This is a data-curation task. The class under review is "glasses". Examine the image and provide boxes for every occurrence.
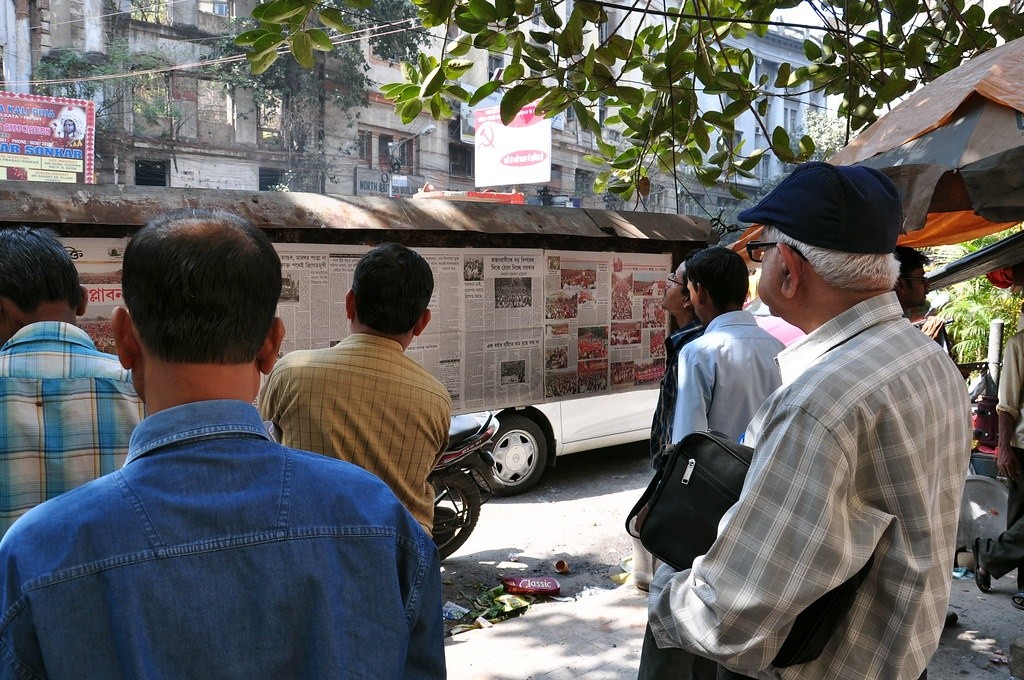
[745,240,810,265]
[899,277,928,284]
[664,272,687,289]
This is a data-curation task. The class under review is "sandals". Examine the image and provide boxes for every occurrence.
[970,536,993,594]
[1011,592,1024,611]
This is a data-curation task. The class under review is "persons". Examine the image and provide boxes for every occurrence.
[650,261,705,470]
[650,248,785,576]
[63,117,77,138]
[971,303,1024,611]
[258,244,451,542]
[892,244,929,314]
[0,226,146,547]
[0,209,449,680]
[637,162,972,680]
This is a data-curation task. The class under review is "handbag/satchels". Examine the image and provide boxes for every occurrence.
[623,429,874,669]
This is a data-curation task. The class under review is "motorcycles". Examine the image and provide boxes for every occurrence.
[969,367,1001,480]
[419,411,502,563]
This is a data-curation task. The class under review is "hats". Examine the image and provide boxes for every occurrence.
[736,164,905,254]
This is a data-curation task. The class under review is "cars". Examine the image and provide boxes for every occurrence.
[445,389,661,497]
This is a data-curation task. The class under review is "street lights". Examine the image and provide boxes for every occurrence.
[389,124,437,198]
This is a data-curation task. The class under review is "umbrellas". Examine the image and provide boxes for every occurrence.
[726,33,1024,269]
